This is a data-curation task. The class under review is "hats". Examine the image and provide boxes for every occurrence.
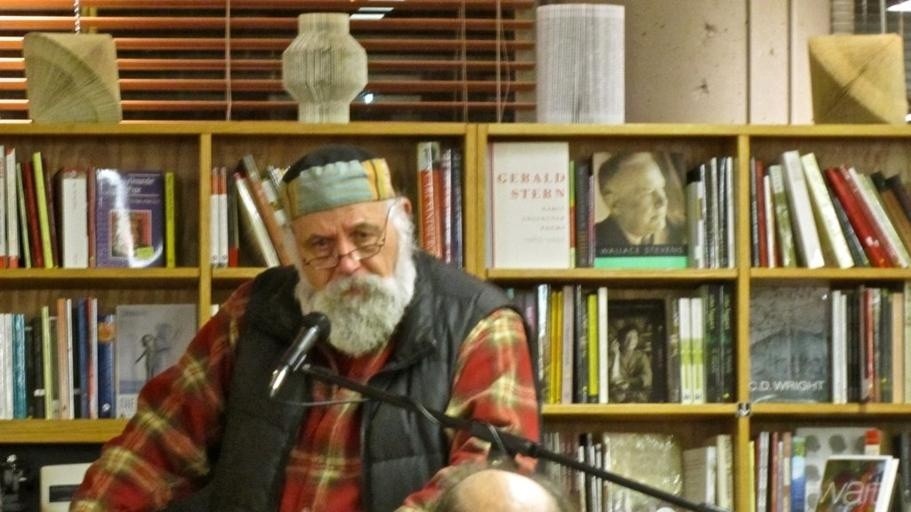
[276,145,396,223]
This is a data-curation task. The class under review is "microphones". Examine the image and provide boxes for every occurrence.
[265,308,331,399]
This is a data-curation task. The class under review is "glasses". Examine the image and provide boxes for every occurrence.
[289,202,399,272]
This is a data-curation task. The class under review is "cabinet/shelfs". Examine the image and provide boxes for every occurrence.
[1,122,910,510]
